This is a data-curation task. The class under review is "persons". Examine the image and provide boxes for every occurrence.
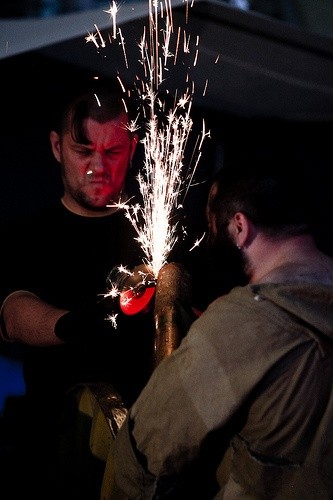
[101,143,333,500]
[0,69,202,500]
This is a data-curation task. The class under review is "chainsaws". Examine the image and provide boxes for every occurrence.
[106,264,157,316]
